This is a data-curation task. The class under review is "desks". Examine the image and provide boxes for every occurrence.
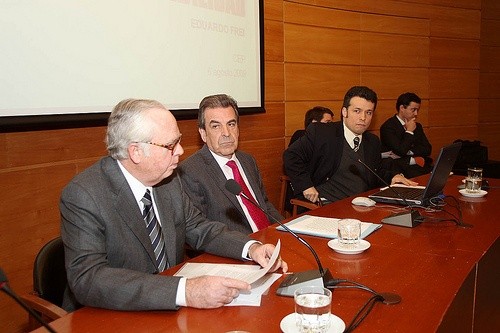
[31,173,500,333]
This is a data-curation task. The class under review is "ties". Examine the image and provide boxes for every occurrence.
[141,189,170,273]
[353,137,360,153]
[226,159,270,230]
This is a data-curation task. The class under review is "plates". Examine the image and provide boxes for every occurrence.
[327,237,371,254]
[459,189,488,197]
[280,311,346,333]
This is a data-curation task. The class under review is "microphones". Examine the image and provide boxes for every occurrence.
[224,178,335,296]
[351,154,421,228]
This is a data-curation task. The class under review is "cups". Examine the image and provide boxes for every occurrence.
[465,167,483,194]
[337,218,362,251]
[294,286,333,333]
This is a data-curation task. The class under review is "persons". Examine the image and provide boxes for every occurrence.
[58,99,288,313]
[282,85,419,216]
[305,106,334,134]
[380,92,434,177]
[179,94,284,257]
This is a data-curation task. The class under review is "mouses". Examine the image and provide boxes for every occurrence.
[351,196,377,207]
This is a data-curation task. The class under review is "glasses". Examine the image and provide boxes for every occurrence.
[132,134,182,156]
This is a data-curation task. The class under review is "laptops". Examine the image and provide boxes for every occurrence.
[368,141,463,207]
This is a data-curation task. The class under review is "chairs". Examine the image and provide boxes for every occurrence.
[279,175,320,218]
[21,237,68,330]
